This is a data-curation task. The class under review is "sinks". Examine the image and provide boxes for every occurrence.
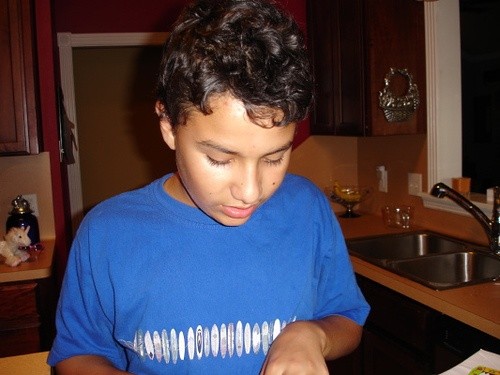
[399,251,500,283]
[345,234,472,261]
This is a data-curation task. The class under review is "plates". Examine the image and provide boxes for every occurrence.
[437,348,500,375]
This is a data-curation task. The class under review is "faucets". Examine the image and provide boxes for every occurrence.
[430,182,492,236]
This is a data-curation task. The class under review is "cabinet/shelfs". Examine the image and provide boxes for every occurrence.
[307,0,425,137]
[0,0,63,151]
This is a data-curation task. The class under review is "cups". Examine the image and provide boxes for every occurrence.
[382,206,396,232]
[396,207,413,229]
